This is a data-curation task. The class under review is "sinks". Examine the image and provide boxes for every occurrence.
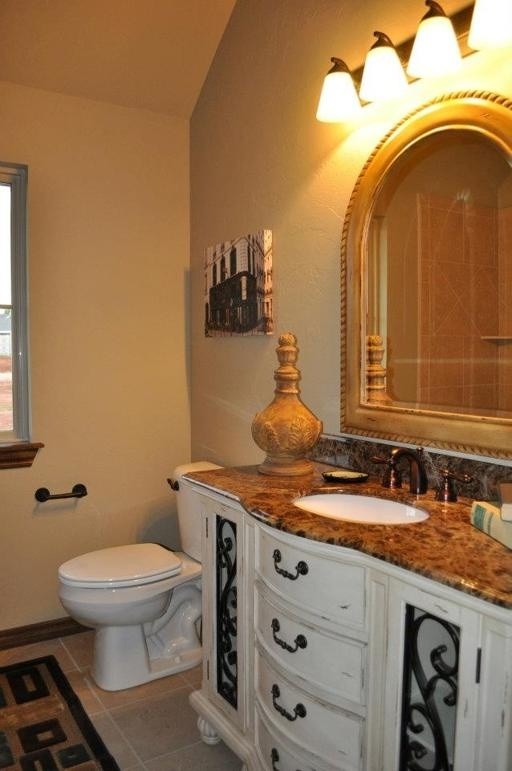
[289,488,430,524]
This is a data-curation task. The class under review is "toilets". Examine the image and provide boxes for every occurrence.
[58,462,225,693]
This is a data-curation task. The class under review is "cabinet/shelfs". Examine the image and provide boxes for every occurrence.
[177,465,512,771]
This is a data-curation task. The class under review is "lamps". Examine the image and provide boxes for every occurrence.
[314,0,511,125]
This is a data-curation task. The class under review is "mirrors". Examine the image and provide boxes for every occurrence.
[339,90,511,460]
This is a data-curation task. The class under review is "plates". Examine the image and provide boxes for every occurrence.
[320,469,370,483]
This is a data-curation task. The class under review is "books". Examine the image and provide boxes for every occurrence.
[497,482,511,522]
[469,500,512,550]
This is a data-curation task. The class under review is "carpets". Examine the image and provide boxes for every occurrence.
[1,655,124,771]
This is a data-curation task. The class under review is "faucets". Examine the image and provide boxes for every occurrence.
[387,447,428,494]
